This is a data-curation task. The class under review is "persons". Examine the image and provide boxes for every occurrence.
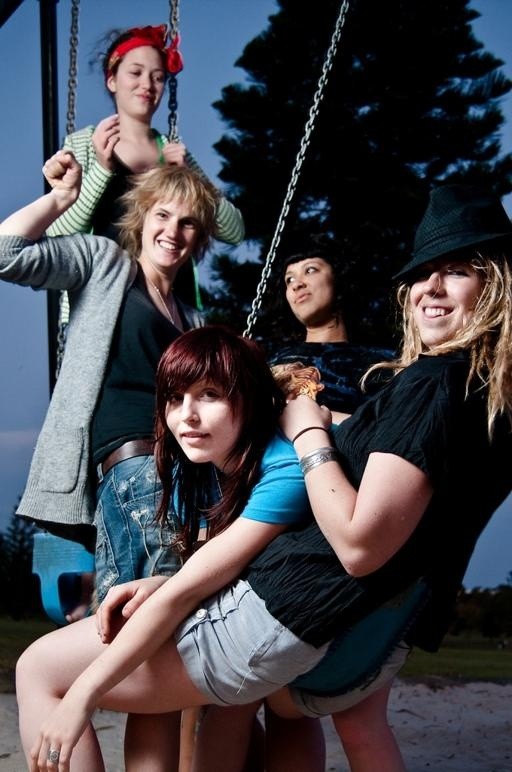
[1,147,226,771]
[200,241,404,772]
[43,25,246,343]
[31,325,413,770]
[15,170,511,772]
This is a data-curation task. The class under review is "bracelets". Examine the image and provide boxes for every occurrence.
[298,446,337,476]
[292,425,327,445]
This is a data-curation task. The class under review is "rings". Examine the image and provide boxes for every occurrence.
[46,749,59,765]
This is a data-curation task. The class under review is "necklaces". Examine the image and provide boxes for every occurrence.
[143,272,178,327]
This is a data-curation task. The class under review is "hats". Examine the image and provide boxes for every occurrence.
[390,174,511,284]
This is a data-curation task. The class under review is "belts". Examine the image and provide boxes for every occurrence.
[96,439,157,477]
